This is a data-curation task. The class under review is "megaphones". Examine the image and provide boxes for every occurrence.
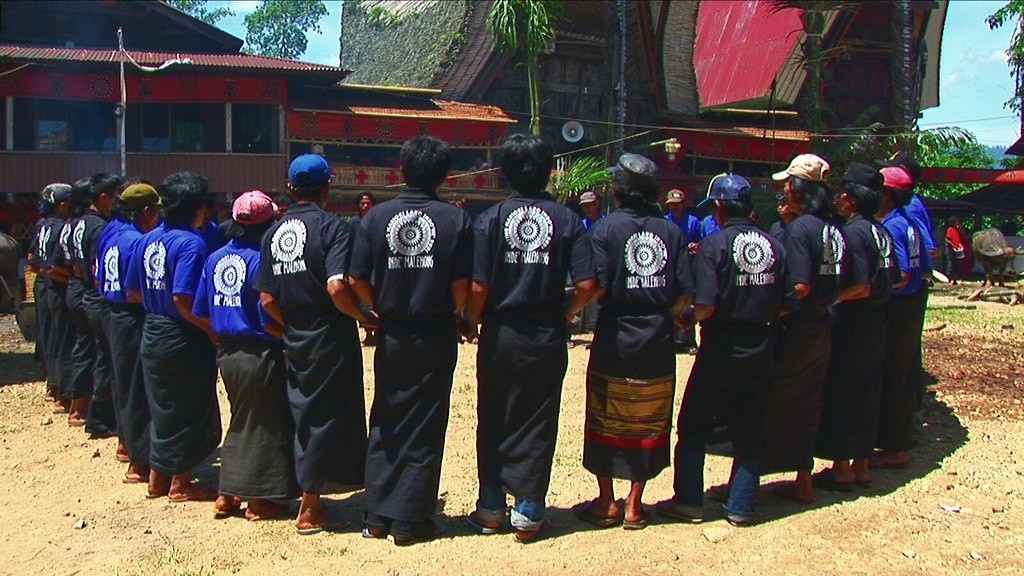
[562,120,584,143]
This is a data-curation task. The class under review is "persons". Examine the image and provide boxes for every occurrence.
[254,151,382,533]
[469,133,599,544]
[348,133,474,548]
[654,172,802,521]
[26,168,298,522]
[569,152,694,530]
[703,152,969,506]
[351,187,702,356]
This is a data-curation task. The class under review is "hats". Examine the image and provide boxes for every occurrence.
[874,151,920,180]
[665,189,685,204]
[578,191,601,205]
[878,166,913,192]
[771,153,832,187]
[120,183,163,209]
[289,153,338,188]
[696,172,752,212]
[605,153,658,193]
[832,162,884,192]
[232,190,279,225]
[38,183,72,215]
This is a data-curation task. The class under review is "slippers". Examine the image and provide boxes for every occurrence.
[45,397,909,547]
